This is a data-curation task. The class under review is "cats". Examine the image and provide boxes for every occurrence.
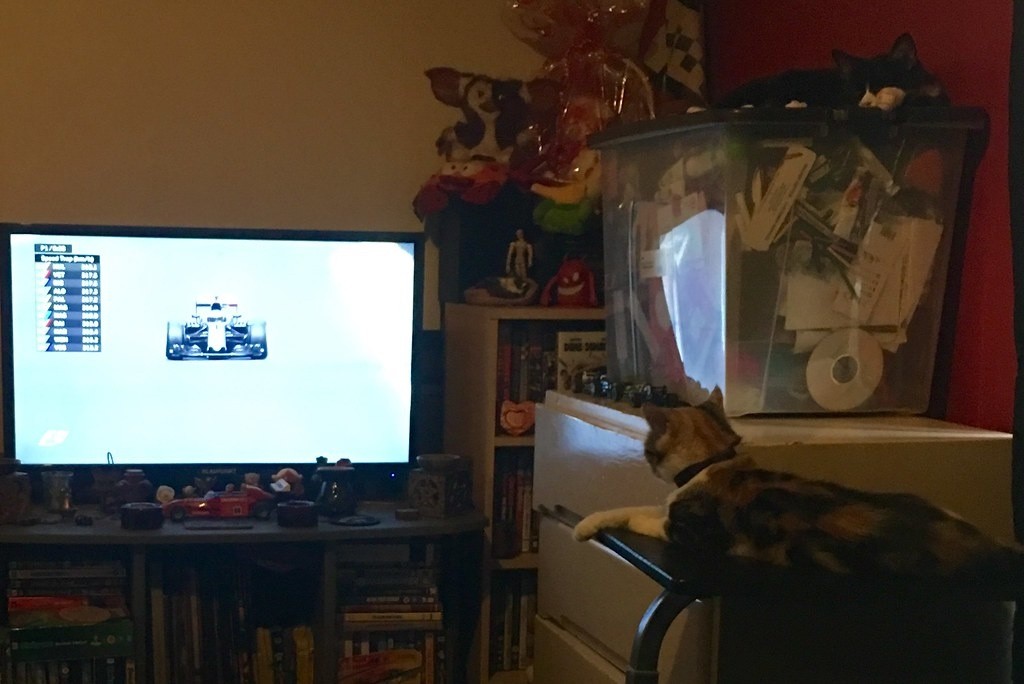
[572,386,1024,601]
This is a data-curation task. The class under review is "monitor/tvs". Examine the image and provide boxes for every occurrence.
[0,223,427,495]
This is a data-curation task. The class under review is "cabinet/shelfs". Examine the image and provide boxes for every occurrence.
[0,497,487,684]
[438,298,623,684]
[520,385,1019,683]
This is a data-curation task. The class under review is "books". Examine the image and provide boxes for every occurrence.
[0,562,442,684]
[489,320,608,671]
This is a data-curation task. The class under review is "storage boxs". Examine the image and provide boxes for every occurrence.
[586,106,990,410]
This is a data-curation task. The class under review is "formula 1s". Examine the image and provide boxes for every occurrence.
[161,483,279,520]
[165,299,269,361]
